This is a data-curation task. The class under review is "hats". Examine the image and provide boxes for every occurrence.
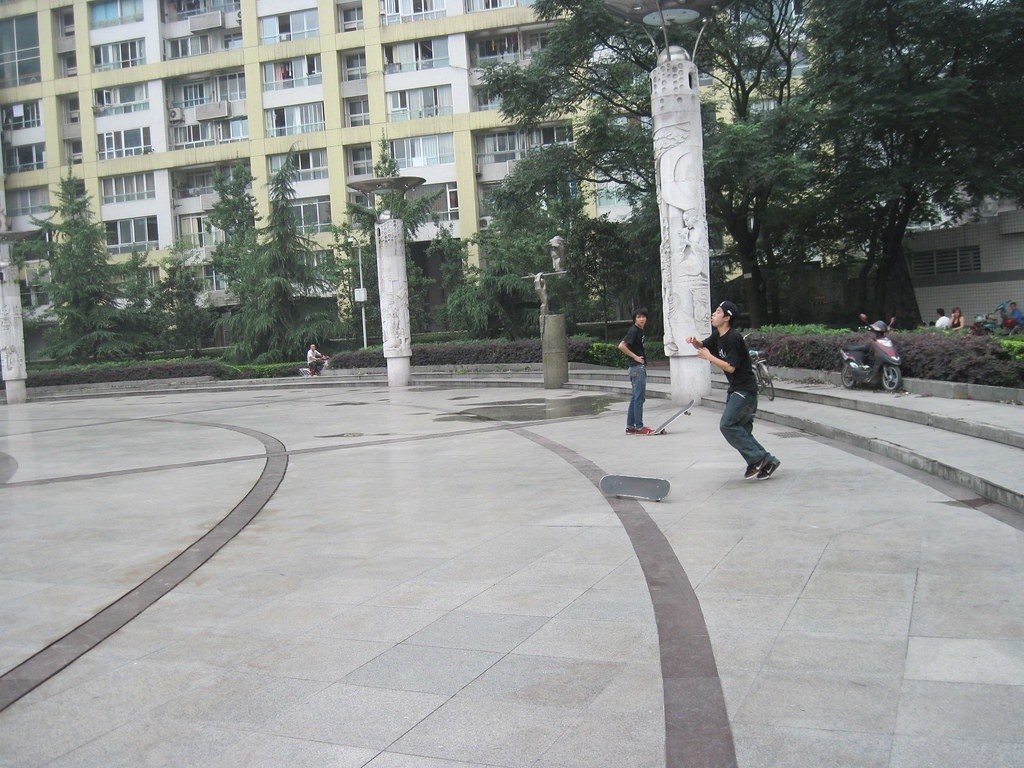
[720,301,740,318]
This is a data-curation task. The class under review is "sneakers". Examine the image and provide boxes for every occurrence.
[743,457,765,480]
[635,426,656,436]
[755,456,781,480]
[625,427,635,435]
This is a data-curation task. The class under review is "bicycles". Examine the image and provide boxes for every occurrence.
[721,334,776,401]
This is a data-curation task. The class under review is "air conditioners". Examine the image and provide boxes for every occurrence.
[168,108,185,122]
[0,271,7,283]
[479,216,492,231]
[236,10,242,21]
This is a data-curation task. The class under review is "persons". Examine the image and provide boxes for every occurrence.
[618,306,655,435]
[935,308,949,329]
[306,344,329,375]
[686,300,781,480]
[929,321,935,325]
[1010,302,1024,325]
[948,306,965,330]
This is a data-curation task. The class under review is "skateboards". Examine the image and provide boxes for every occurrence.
[654,399,695,435]
[598,475,672,502]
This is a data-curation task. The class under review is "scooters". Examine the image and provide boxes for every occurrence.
[299,354,330,377]
[970,300,1024,339]
[840,313,902,391]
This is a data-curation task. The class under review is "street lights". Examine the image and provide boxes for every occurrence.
[347,176,427,388]
[603,0,732,409]
[342,229,369,352]
